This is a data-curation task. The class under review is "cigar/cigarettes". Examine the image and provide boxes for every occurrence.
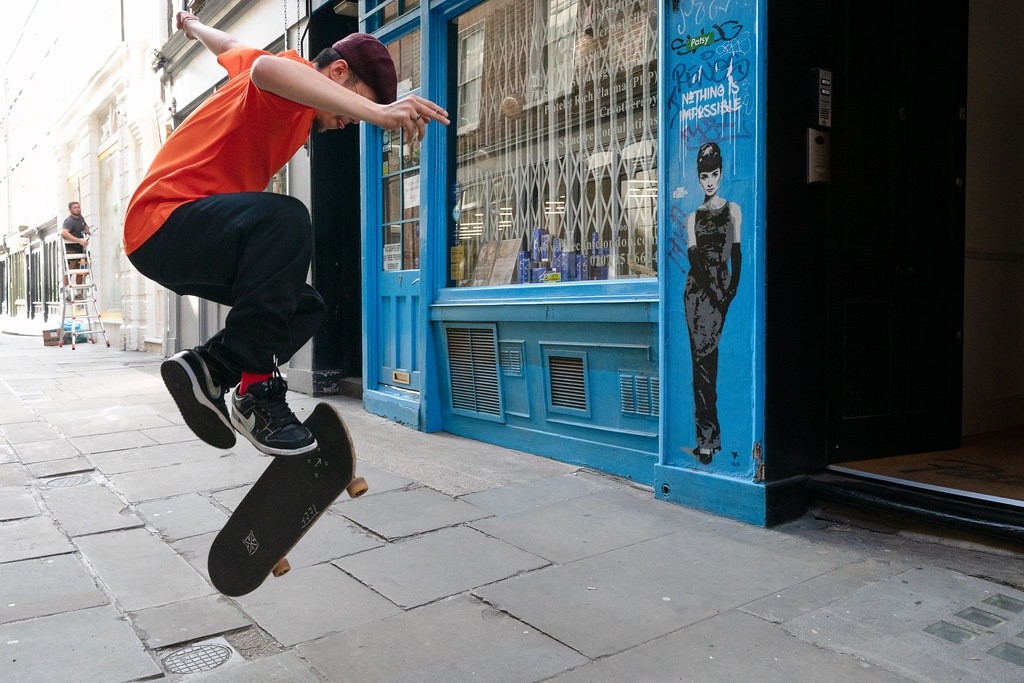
[435,110,440,114]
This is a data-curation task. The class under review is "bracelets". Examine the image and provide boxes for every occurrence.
[181,16,200,41]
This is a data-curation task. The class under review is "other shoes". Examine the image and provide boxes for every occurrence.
[692,446,712,465]
[66,295,71,301]
[74,294,86,300]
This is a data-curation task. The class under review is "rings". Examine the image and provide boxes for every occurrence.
[412,113,422,122]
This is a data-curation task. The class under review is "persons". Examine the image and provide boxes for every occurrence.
[682,143,743,474]
[61,200,92,303]
[123,11,451,458]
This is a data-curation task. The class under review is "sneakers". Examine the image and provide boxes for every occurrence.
[229,366,319,457]
[161,343,237,450]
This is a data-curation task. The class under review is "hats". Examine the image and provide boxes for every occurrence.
[332,32,397,105]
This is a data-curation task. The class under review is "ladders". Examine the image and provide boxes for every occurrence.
[58,232,110,350]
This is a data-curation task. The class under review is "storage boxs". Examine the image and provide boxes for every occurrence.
[43,329,62,346]
